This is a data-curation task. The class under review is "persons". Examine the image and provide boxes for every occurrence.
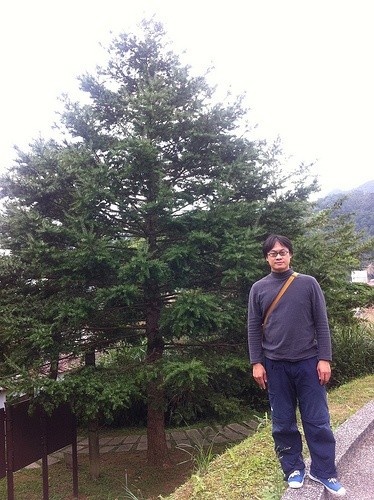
[247,235,346,497]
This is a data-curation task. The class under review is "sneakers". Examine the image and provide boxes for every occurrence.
[308,470,346,496]
[288,469,306,488]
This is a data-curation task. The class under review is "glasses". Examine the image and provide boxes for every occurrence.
[267,250,288,256]
